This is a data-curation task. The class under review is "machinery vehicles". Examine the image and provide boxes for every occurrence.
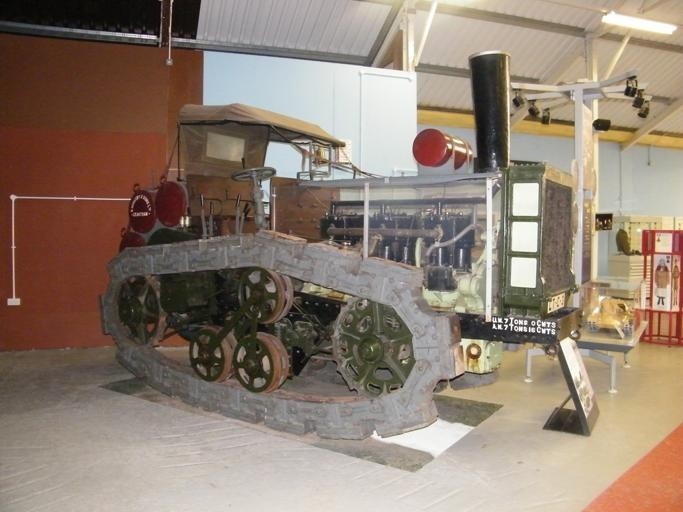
[99,50,583,446]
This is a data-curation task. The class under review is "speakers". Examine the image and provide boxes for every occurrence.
[593,119,611,131]
